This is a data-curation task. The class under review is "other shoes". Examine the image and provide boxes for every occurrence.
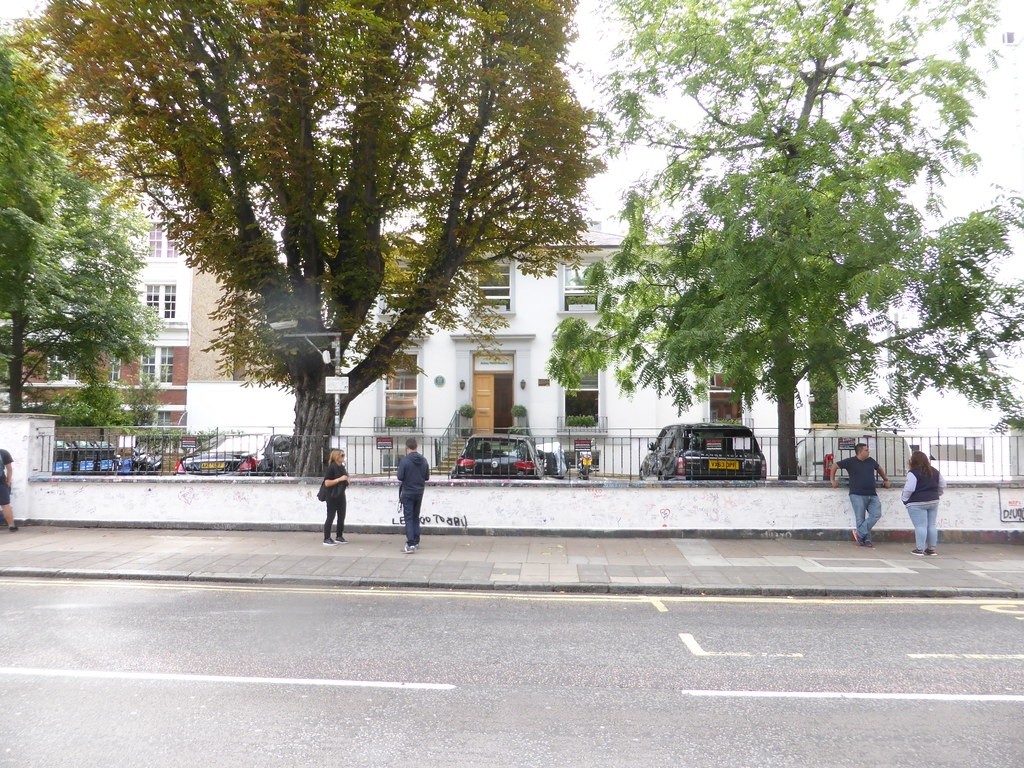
[9,525,18,532]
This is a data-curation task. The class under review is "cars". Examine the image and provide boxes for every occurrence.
[638,423,767,481]
[172,434,293,476]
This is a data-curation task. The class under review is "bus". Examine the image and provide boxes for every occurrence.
[450,434,545,480]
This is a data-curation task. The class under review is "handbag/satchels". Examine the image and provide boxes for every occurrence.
[317,481,329,501]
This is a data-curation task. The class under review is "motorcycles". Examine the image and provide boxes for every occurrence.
[132,442,163,475]
[575,438,595,480]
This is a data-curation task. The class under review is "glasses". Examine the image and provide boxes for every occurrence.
[339,454,345,457]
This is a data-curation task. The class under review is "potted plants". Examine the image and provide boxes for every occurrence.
[508,405,527,434]
[459,405,476,436]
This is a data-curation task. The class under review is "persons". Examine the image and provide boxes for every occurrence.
[397,437,430,553]
[901,451,947,556]
[830,443,890,547]
[0,449,18,532]
[323,449,350,546]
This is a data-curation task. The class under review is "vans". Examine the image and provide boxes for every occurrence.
[796,424,911,479]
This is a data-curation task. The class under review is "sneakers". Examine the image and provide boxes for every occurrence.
[924,548,937,555]
[852,529,865,546]
[911,548,924,556]
[413,544,419,552]
[400,545,415,554]
[862,537,873,547]
[323,538,338,546]
[334,536,349,544]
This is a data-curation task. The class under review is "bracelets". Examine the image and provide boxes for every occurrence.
[884,478,888,481]
[336,478,339,483]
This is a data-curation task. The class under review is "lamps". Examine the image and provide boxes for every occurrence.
[460,380,465,390]
[521,379,526,389]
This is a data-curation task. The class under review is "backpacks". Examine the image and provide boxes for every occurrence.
[0,456,4,481]
